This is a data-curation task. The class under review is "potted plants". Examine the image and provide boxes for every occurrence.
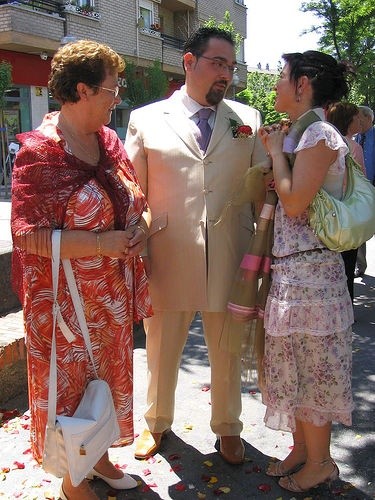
[77,4,99,18]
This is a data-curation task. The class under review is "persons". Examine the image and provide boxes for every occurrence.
[9,39,152,500]
[245,51,354,491]
[261,102,374,304]
[120,26,271,463]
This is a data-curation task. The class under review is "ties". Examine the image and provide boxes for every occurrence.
[193,109,212,156]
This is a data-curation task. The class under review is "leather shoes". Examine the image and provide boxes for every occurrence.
[134,429,163,459]
[218,435,246,464]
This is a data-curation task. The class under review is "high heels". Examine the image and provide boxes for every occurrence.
[86,467,137,489]
[278,457,340,493]
[59,483,99,500]
[266,443,307,476]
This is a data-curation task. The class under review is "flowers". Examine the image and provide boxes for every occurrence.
[230,119,253,142]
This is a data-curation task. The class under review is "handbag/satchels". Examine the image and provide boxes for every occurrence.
[305,156,375,253]
[42,379,121,488]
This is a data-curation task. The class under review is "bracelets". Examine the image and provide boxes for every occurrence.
[95,232,100,257]
[137,225,146,236]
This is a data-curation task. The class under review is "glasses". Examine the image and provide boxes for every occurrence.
[92,84,119,97]
[197,54,239,73]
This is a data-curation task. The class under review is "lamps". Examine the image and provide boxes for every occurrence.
[40,50,47,60]
